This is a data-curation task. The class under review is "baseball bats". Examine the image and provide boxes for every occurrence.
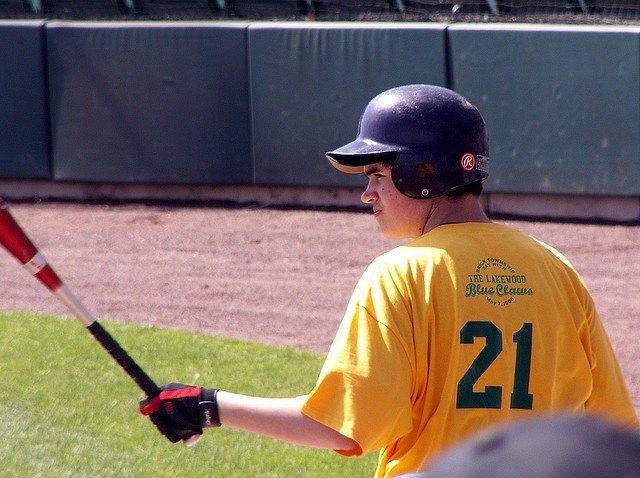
[0,195,202,448]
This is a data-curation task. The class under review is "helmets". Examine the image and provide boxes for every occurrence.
[325,83,490,198]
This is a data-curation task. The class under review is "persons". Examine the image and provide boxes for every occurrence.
[139,83,640,478]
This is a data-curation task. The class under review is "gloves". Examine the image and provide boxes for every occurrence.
[138,383,222,443]
